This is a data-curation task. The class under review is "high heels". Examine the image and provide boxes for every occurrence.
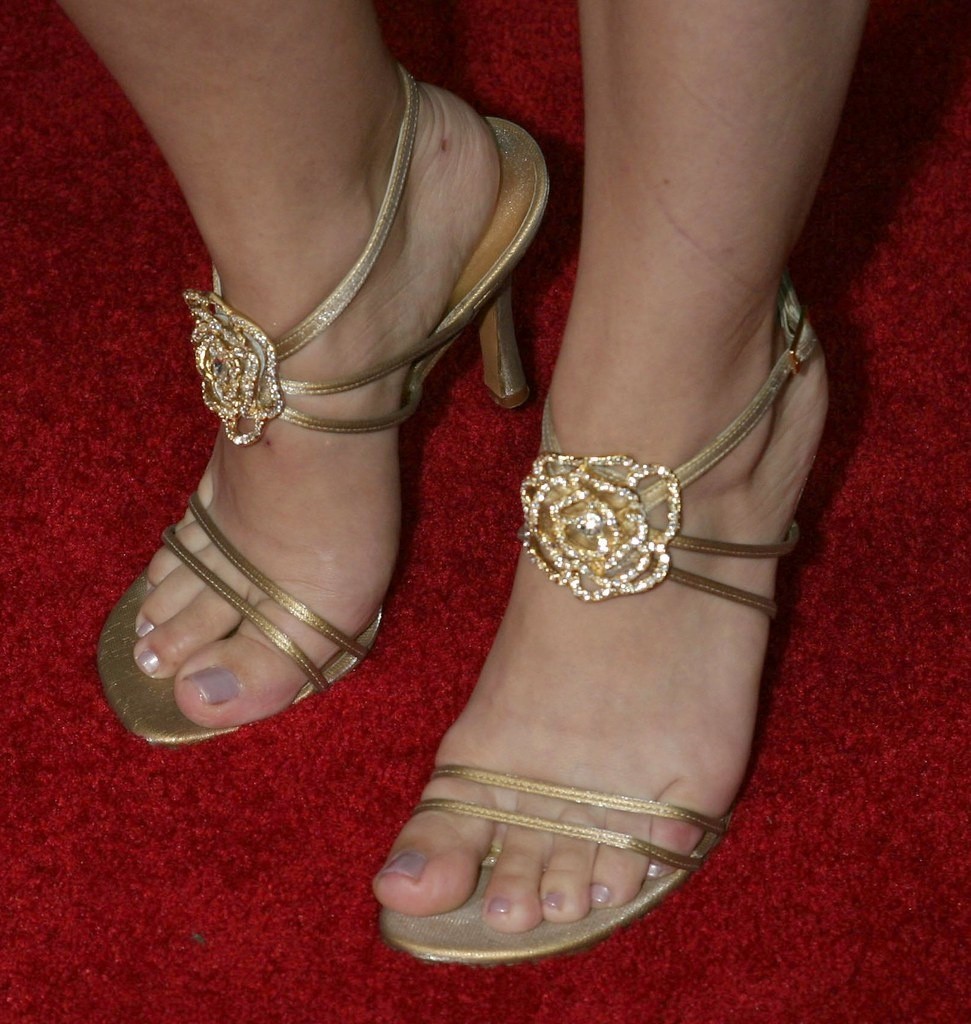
[97,59,550,743]
[380,277,810,964]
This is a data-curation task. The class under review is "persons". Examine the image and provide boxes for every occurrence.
[53,1,869,965]
